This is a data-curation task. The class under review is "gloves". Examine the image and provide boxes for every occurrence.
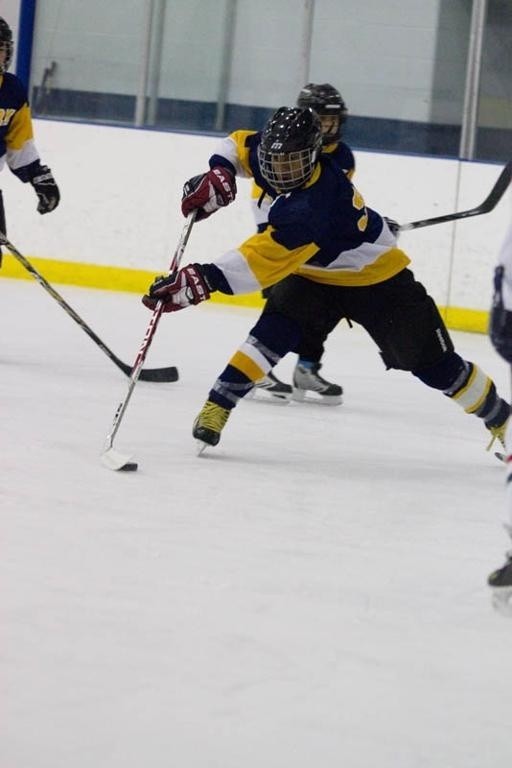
[142,264,211,313]
[31,165,60,214]
[181,164,237,222]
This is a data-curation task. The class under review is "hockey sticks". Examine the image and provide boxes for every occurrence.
[400,161,512,231]
[1,233,178,383]
[100,207,197,472]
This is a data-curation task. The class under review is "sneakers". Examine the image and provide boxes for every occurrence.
[192,363,343,447]
[486,414,512,484]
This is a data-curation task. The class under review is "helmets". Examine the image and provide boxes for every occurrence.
[0,17,13,75]
[257,83,348,191]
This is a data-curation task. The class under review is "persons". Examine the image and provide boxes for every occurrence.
[140,106,512,484]
[247,82,356,396]
[486,221,512,587]
[0,13,59,276]
